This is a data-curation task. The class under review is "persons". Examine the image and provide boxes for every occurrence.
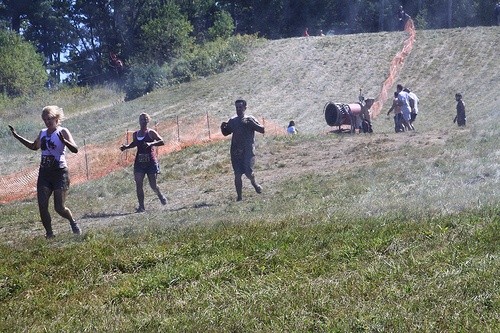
[221,99,264,202]
[120,111,167,213]
[387,84,419,132]
[359,99,374,133]
[453,92,467,128]
[287,120,298,135]
[8,105,81,238]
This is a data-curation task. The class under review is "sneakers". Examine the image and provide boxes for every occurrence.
[46,232,55,240]
[70,218,81,236]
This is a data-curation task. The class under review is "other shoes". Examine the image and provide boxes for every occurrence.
[158,193,167,205]
[135,207,144,212]
[255,185,262,194]
[237,197,242,201]
